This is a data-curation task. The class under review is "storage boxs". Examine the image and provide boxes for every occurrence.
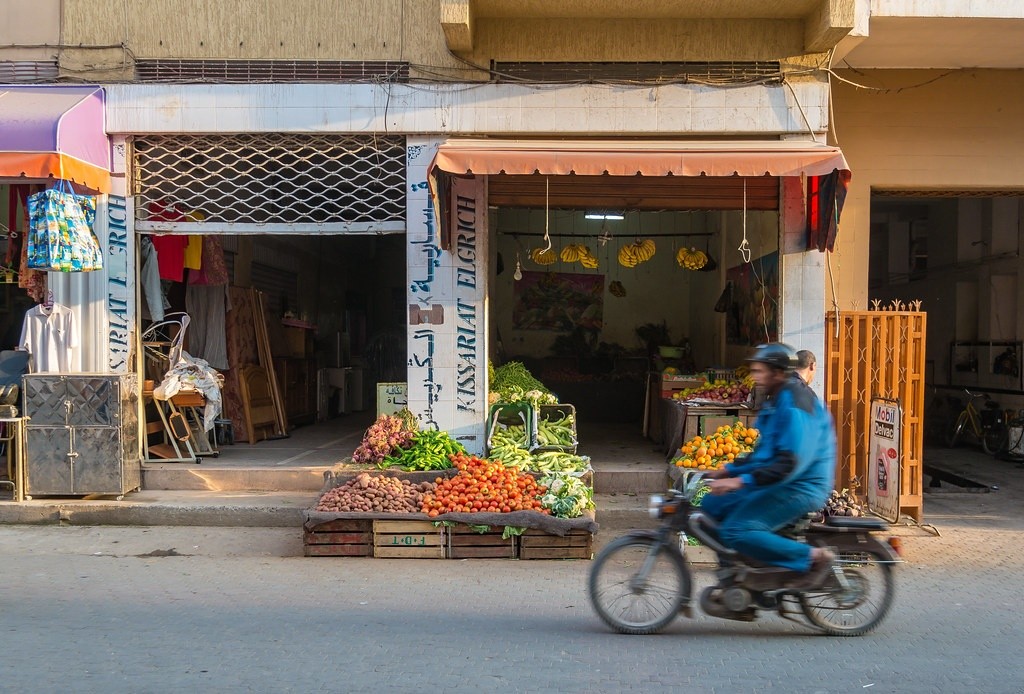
[304,403,596,559]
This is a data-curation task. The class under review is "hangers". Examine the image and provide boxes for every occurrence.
[0,222,18,239]
[42,291,54,306]
[0,261,19,283]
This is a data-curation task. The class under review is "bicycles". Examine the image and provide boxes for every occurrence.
[950,387,1011,456]
[924,384,967,448]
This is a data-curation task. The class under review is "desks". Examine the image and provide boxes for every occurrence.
[0,418,24,502]
[650,381,757,446]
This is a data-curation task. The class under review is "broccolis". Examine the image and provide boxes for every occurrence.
[545,394,556,404]
[526,389,543,401]
[489,392,501,404]
[536,473,594,518]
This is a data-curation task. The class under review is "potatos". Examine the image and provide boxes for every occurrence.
[314,473,438,514]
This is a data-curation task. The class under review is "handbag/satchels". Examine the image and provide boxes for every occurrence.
[26,177,103,275]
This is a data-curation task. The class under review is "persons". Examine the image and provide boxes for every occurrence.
[698,343,836,594]
[792,350,818,399]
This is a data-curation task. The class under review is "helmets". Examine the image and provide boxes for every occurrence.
[744,342,800,369]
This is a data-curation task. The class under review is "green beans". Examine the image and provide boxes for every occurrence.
[686,485,712,546]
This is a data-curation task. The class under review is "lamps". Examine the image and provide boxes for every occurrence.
[584,211,625,220]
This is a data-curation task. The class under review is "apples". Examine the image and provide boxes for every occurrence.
[673,379,750,403]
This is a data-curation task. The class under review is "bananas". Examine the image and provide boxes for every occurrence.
[676,246,708,271]
[617,239,656,268]
[742,373,756,390]
[531,241,600,268]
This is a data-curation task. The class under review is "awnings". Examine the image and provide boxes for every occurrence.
[427,137,853,254]
[0,86,112,194]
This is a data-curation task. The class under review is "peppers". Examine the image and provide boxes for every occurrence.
[491,360,550,404]
[488,410,590,475]
[376,426,475,472]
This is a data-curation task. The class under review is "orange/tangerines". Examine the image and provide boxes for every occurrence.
[674,421,759,471]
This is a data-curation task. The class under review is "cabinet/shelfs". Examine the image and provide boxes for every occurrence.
[22,371,142,500]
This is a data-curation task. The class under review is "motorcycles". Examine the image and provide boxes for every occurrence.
[587,471,902,638]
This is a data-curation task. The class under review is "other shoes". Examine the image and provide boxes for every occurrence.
[800,549,834,592]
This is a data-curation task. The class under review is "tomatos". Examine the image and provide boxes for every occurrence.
[419,452,552,518]
